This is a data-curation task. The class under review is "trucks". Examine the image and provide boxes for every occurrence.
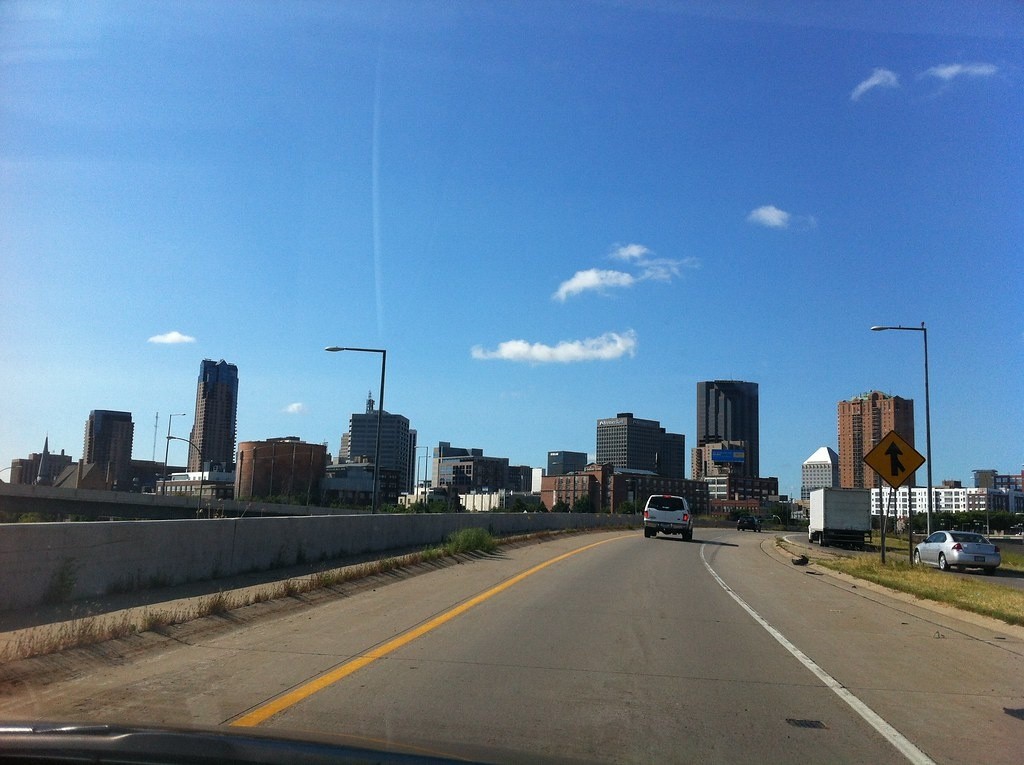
[808,487,872,549]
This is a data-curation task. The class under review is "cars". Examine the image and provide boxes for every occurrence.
[913,530,1002,575]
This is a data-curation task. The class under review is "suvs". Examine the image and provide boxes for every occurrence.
[644,494,693,542]
[737,516,761,533]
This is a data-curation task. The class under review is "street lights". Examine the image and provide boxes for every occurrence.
[162,413,186,496]
[871,323,932,537]
[552,462,577,513]
[166,436,204,518]
[325,346,388,515]
[414,445,432,513]
